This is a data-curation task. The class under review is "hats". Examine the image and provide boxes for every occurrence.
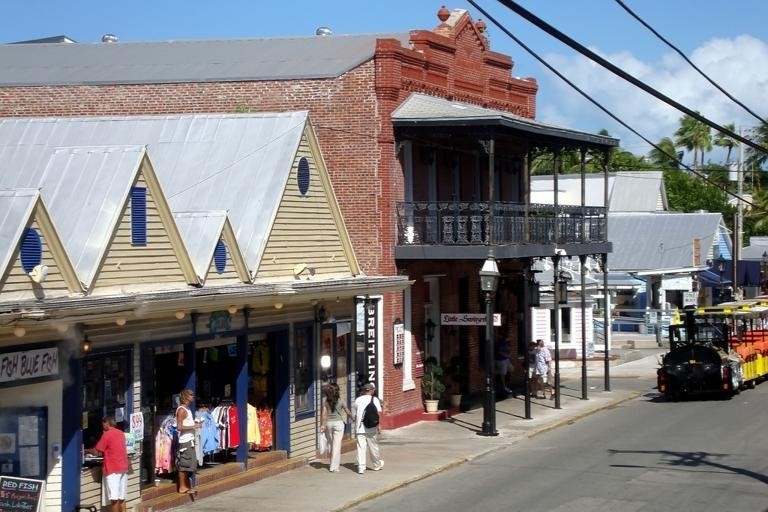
[363,384,375,390]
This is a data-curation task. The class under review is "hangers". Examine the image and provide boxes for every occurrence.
[195,398,256,418]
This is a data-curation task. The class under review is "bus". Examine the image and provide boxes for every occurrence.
[659,295,768,401]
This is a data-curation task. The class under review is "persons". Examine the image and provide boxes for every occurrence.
[320,383,355,472]
[84,416,128,512]
[175,390,202,494]
[352,384,384,474]
[527,340,553,399]
[497,343,516,392]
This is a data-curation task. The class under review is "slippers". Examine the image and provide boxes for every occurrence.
[181,488,197,494]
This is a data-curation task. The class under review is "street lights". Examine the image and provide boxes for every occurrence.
[477,250,501,435]
[734,203,752,293]
[717,255,726,302]
[762,250,768,295]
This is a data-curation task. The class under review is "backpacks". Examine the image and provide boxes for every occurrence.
[363,403,379,428]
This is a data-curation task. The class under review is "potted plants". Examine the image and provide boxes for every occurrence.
[421,353,467,414]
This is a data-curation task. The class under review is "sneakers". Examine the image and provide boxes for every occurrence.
[358,469,365,474]
[373,460,385,471]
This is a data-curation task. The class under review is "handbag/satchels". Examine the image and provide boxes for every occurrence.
[340,409,348,425]
[547,371,556,388]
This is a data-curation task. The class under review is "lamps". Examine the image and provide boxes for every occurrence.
[528,255,568,307]
[296,266,316,276]
[228,304,238,315]
[80,339,92,353]
[115,318,127,326]
[274,303,284,309]
[424,318,436,342]
[174,310,185,319]
[13,326,26,338]
